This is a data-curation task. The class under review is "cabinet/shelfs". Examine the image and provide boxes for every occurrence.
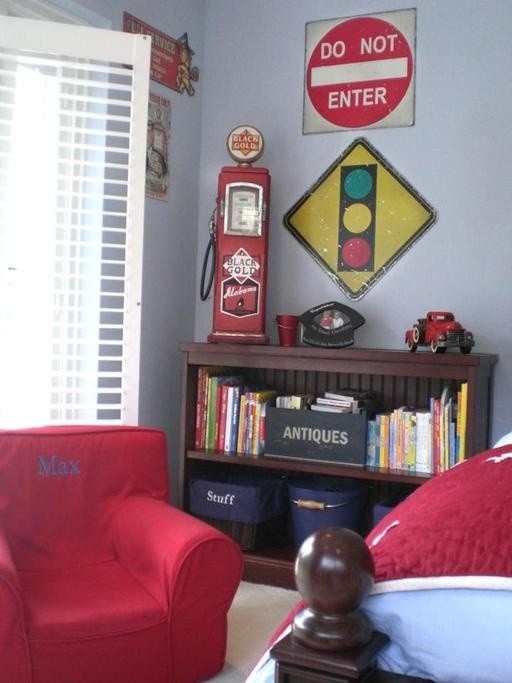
[178,342,499,591]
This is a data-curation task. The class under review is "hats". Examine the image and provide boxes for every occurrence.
[176,32,195,55]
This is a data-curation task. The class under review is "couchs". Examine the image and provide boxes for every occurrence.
[0,424,244,683]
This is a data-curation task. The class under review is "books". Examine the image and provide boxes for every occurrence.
[196,365,467,475]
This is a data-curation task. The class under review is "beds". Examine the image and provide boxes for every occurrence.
[244,432,512,683]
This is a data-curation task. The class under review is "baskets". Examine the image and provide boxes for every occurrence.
[189,472,288,551]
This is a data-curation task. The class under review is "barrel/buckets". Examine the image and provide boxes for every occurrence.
[287,479,368,550]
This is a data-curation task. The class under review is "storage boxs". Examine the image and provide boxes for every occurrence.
[264,402,385,470]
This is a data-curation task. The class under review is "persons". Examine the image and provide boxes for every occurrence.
[176,32,196,95]
[321,310,345,329]
